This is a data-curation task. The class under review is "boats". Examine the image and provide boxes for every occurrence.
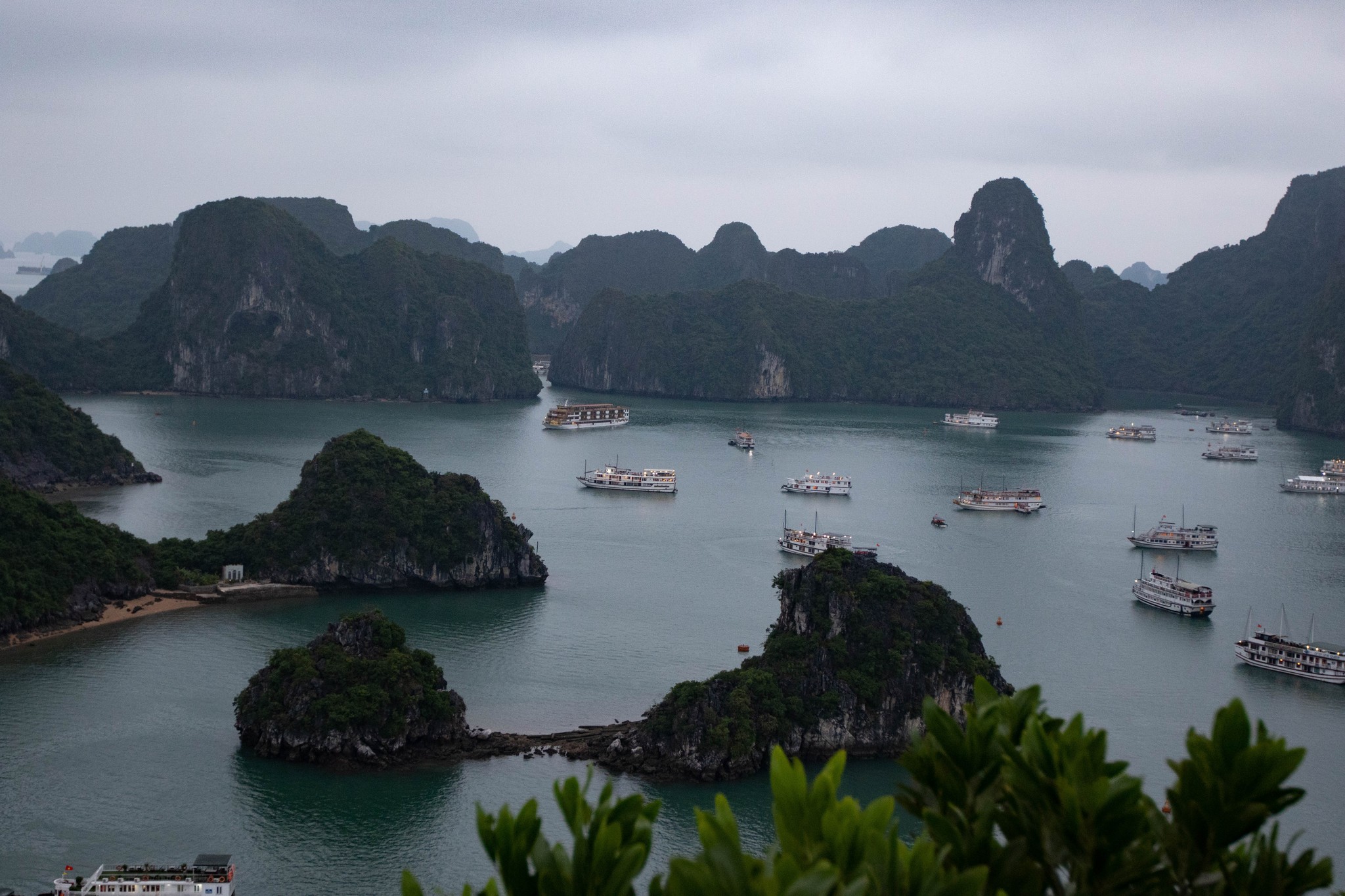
[532,360,551,375]
[1015,502,1032,514]
[1206,417,1252,434]
[1235,602,1345,685]
[1106,424,1156,441]
[940,408,1000,429]
[1132,549,1217,619]
[778,509,852,559]
[734,417,755,449]
[1171,402,1215,418]
[953,472,1043,511]
[37,853,238,896]
[1261,426,1269,431]
[852,544,879,557]
[1201,443,1259,461]
[781,469,853,497]
[1278,457,1345,494]
[931,513,948,527]
[542,398,629,430]
[576,454,678,493]
[727,438,736,445]
[1126,503,1219,551]
